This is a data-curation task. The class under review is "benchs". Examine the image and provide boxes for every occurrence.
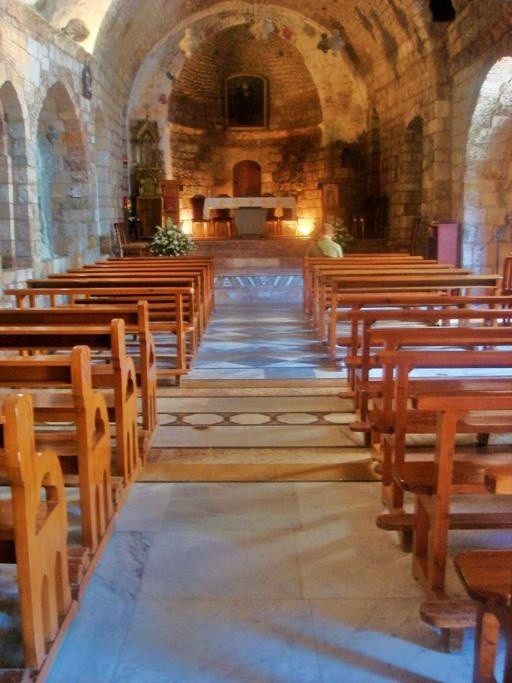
[302,253,511,681]
[0,255,217,675]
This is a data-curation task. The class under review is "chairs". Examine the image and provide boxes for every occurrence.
[115,222,149,258]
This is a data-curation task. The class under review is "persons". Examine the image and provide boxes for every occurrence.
[305,223,344,257]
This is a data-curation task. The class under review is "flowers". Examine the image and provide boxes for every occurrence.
[144,218,198,257]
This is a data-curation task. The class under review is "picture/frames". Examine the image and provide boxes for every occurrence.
[224,74,268,131]
